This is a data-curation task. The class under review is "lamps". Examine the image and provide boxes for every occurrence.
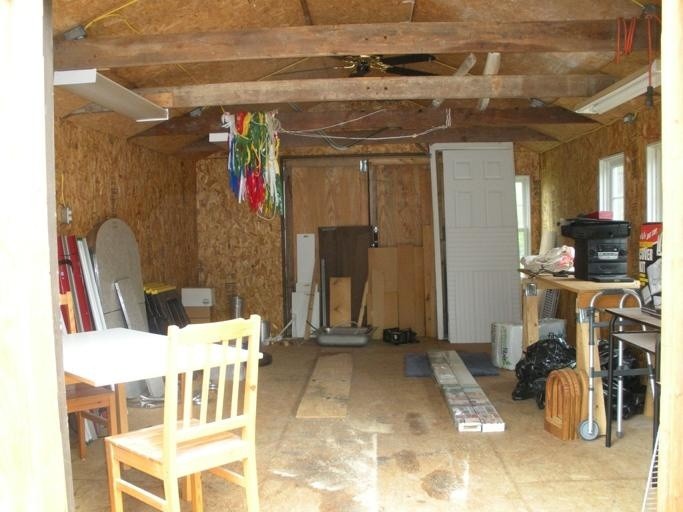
[575,59,661,115]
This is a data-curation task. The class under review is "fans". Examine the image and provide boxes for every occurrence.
[329,55,437,77]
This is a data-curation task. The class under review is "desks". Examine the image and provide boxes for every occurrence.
[63,328,263,502]
[518,268,641,436]
[605,307,661,488]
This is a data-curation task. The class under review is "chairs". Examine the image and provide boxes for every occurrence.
[59,292,118,460]
[105,314,259,512]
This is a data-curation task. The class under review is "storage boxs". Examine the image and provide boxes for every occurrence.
[184,306,215,324]
[181,287,216,307]
